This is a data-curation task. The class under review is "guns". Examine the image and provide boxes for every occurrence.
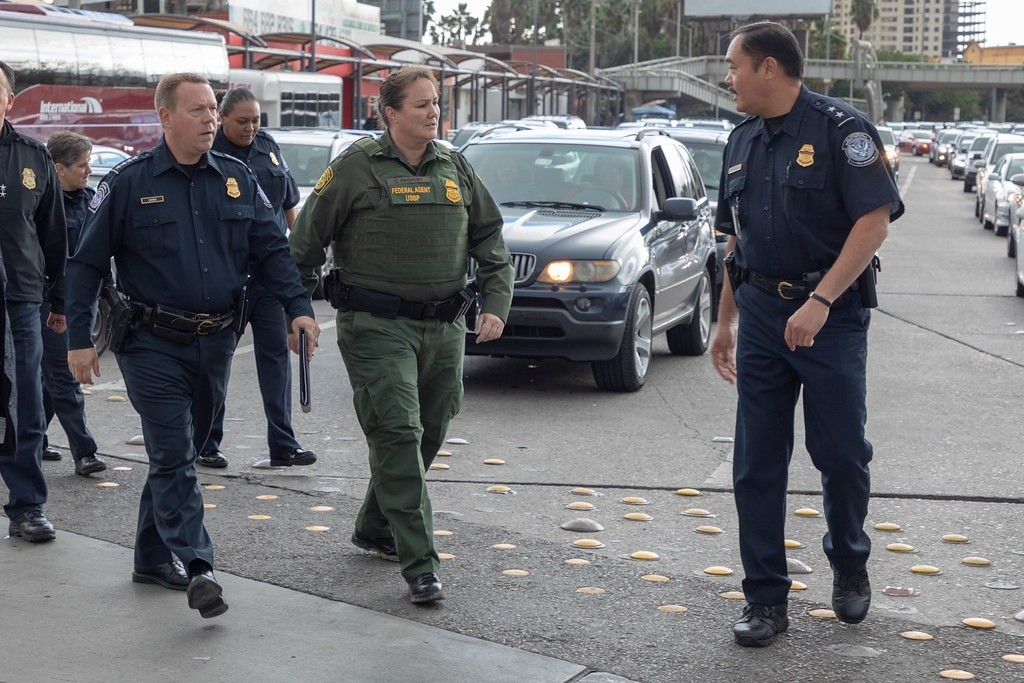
[467,279,480,333]
[725,252,745,309]
[101,284,132,352]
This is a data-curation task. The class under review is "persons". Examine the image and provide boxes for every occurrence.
[38,129,112,475]
[66,73,321,619]
[708,21,906,646]
[195,88,317,467]
[0,60,69,542]
[595,154,633,208]
[287,65,516,602]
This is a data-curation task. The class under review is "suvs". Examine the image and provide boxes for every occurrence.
[451,124,719,391]
[262,116,737,320]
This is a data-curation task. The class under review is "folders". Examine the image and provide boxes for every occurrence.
[298,326,311,413]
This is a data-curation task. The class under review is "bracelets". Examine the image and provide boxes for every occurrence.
[809,292,832,308]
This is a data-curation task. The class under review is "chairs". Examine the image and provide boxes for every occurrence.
[483,155,536,201]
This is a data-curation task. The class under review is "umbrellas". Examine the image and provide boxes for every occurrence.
[634,104,675,115]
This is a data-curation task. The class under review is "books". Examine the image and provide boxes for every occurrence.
[298,327,312,413]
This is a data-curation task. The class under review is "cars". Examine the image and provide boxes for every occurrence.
[875,121,1024,297]
[43,143,135,359]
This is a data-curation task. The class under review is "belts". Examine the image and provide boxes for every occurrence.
[748,267,808,301]
[398,297,452,320]
[133,302,237,336]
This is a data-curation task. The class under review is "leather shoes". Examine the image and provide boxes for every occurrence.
[269,444,318,466]
[8,505,55,541]
[732,597,789,646]
[197,448,229,467]
[186,564,228,619]
[41,446,62,460]
[405,570,445,605]
[74,452,107,475]
[832,562,872,623]
[351,526,399,561]
[132,561,190,591]
